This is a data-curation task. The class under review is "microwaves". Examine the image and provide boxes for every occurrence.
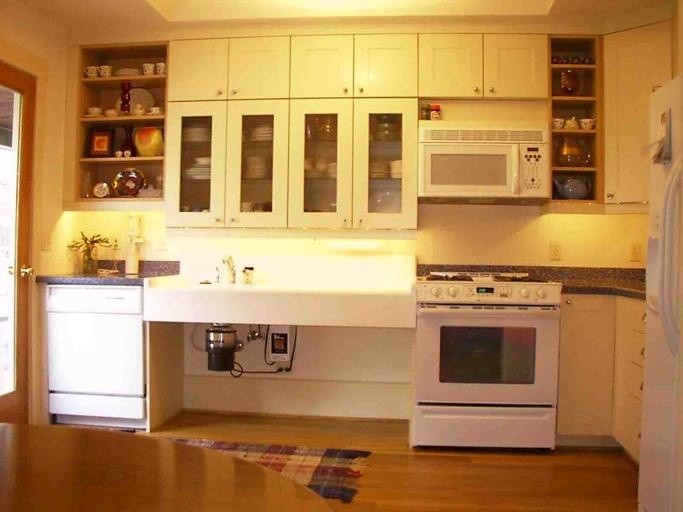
[418,120,553,208]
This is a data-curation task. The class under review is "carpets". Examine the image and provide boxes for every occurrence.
[162,433,370,506]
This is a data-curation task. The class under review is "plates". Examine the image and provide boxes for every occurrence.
[93,182,108,199]
[112,168,145,197]
[182,116,402,212]
[114,88,153,113]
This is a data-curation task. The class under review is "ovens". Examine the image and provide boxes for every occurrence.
[411,305,559,451]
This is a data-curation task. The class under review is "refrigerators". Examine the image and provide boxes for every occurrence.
[641,73,683,408]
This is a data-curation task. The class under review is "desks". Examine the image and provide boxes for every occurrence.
[1,422,338,512]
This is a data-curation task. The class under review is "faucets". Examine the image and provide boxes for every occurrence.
[222,256,238,284]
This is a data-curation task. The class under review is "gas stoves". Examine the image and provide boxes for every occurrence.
[418,268,563,304]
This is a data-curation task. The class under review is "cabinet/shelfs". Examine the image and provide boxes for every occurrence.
[166,99,289,231]
[558,289,613,451]
[546,35,604,216]
[74,43,166,204]
[613,288,646,463]
[288,99,418,233]
[602,18,674,202]
[418,35,547,100]
[167,35,289,100]
[290,33,419,97]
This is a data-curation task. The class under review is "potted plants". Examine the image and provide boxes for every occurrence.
[65,230,111,277]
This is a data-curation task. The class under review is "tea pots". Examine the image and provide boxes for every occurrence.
[554,175,594,200]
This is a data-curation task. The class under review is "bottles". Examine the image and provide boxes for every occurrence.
[420,104,429,120]
[431,104,440,120]
[119,126,135,158]
[554,134,591,166]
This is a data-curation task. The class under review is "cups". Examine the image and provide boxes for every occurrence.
[579,120,593,129]
[83,63,164,77]
[551,118,564,129]
[79,106,162,118]
[132,126,162,158]
[564,119,577,129]
[552,55,593,64]
